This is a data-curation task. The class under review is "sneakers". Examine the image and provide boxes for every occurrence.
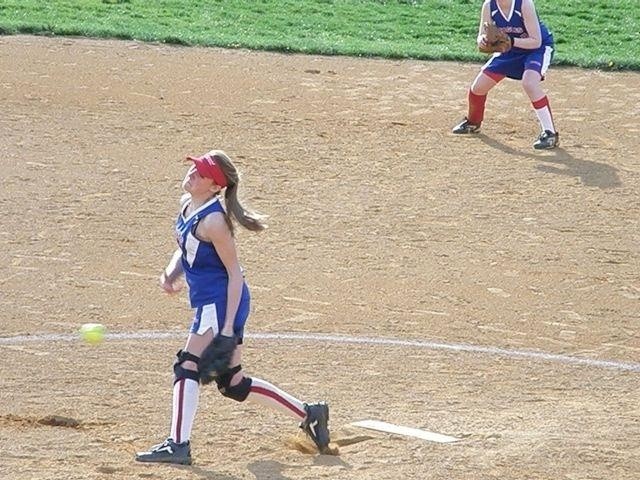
[135,437,193,466]
[452,117,482,135]
[532,129,560,149]
[299,401,330,451]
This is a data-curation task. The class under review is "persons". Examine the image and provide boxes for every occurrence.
[453,0,559,149]
[137,150,331,466]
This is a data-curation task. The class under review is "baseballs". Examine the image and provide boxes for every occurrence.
[81,323,104,343]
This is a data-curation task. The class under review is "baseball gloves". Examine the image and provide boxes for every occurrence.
[197,332,238,385]
[479,21,512,53]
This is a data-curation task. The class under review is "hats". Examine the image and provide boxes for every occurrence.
[185,153,229,189]
